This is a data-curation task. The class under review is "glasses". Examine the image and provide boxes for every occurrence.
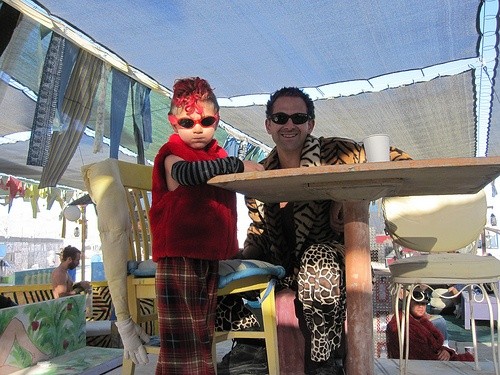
[267,112,312,125]
[168,113,219,129]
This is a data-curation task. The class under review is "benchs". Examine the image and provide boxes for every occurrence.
[0,294,125,375]
[461,279,500,330]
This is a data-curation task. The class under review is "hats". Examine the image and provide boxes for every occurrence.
[406,287,431,304]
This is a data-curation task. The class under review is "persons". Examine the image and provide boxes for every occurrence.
[214,87,414,375]
[52,245,92,299]
[148,78,265,375]
[386,291,475,362]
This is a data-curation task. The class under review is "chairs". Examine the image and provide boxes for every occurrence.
[381,189,500,375]
[82,159,285,375]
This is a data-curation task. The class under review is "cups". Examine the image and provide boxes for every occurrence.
[362,134,390,162]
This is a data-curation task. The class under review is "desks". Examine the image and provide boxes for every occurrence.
[207,156,500,375]
